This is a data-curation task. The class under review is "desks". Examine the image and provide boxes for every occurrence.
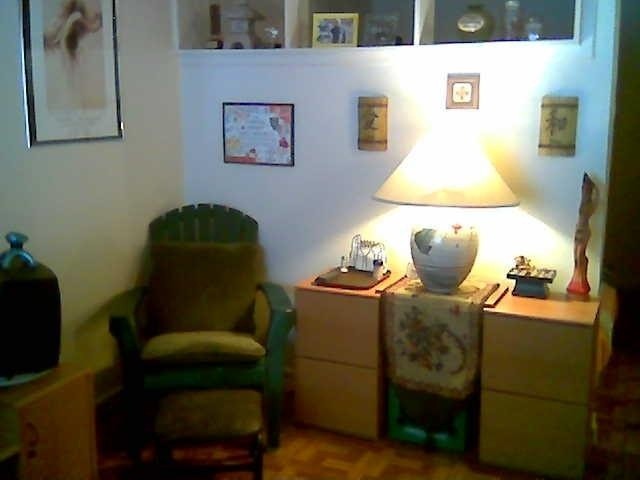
[296,262,602,477]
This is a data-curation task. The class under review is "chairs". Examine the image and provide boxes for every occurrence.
[110,203,294,447]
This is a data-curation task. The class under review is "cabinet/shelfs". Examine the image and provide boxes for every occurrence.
[170,0,583,64]
[0,366,98,480]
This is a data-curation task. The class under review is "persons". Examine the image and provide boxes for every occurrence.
[329,18,348,44]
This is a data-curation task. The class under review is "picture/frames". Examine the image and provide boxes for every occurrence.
[446,76,479,108]
[312,12,359,49]
[19,0,125,149]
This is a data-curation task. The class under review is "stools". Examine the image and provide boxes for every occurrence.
[154,387,268,479]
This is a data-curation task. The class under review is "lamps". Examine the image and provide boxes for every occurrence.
[374,132,519,294]
